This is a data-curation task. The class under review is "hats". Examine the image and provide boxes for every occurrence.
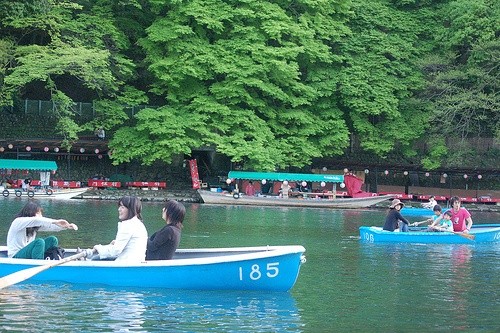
[389,199,404,210]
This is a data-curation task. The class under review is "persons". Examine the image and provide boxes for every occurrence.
[0,286,179,333]
[77,200,186,261]
[421,195,472,234]
[97,126,105,141]
[93,173,106,190]
[389,244,472,277]
[0,175,31,192]
[94,127,100,141]
[245,181,255,197]
[383,199,410,232]
[91,196,147,261]
[7,200,78,260]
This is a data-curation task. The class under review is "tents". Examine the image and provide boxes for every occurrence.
[227,170,344,183]
[0,159,58,171]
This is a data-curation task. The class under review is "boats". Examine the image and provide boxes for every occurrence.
[358,221,500,244]
[196,170,395,209]
[0,242,307,293]
[0,159,94,200]
[397,205,451,218]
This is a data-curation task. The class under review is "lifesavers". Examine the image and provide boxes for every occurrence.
[233,192,239,199]
[3,190,9,197]
[28,191,34,198]
[15,190,22,197]
[46,188,53,195]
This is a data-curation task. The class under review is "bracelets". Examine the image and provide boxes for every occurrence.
[466,228,470,231]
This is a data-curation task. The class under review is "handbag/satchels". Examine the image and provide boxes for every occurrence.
[43,246,65,260]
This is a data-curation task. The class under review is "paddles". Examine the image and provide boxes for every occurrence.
[428,225,476,240]
[411,218,432,226]
[0,243,101,288]
